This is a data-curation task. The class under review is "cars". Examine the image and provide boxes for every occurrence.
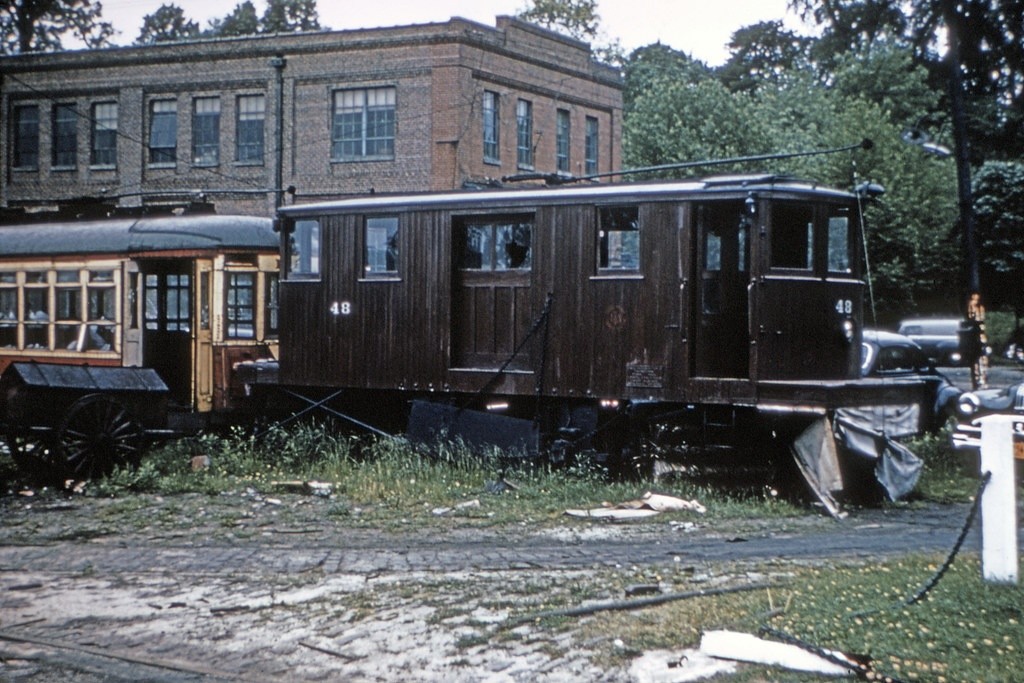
[951,382,1024,462]
[897,319,970,367]
[861,330,965,431]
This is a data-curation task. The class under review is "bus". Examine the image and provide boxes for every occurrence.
[0,216,319,435]
[239,173,938,499]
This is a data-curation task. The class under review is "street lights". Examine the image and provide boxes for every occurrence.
[922,141,954,158]
